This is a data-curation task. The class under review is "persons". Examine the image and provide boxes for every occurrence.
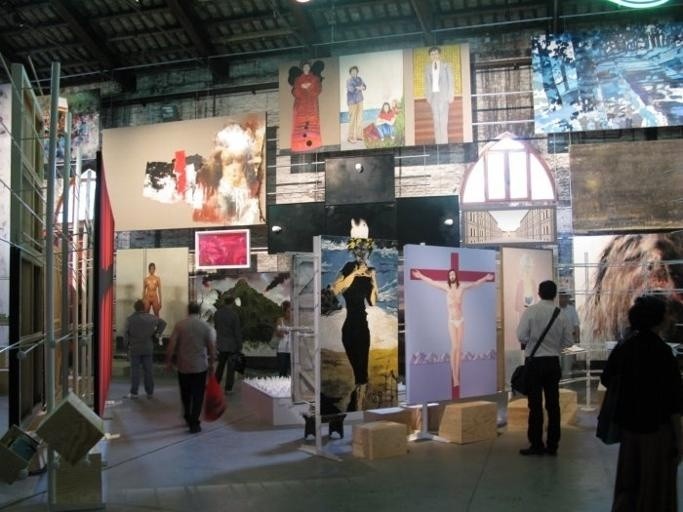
[424,47,455,145]
[141,263,164,346]
[584,232,683,347]
[330,245,378,411]
[412,269,493,386]
[517,281,582,456]
[291,63,322,152]
[346,66,399,145]
[271,300,291,378]
[124,295,245,436]
[194,128,264,223]
[596,295,683,512]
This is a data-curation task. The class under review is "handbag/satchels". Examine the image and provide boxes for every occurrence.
[230,351,246,373]
[511,366,531,396]
[596,385,620,444]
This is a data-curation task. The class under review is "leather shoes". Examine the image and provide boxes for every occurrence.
[520,446,544,456]
[547,441,558,454]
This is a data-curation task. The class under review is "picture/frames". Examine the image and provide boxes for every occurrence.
[194,229,251,271]
